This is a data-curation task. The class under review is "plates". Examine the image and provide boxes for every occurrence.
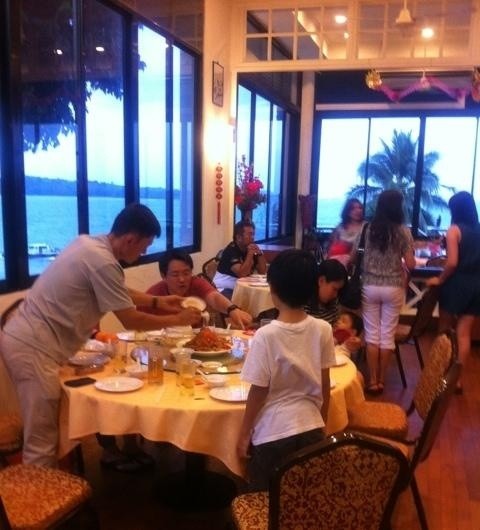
[180,296,207,312]
[332,355,348,366]
[191,359,202,367]
[176,338,233,358]
[202,361,222,369]
[115,331,148,342]
[330,380,336,389]
[94,376,144,392]
[209,385,249,402]
[69,349,112,365]
[194,326,228,335]
[250,273,268,278]
[146,330,166,343]
[248,282,269,287]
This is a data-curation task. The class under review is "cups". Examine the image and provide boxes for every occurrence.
[175,353,190,387]
[178,361,196,397]
[260,318,272,327]
[112,341,128,374]
[148,345,164,386]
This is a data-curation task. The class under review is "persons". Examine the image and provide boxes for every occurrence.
[426,192,480,393]
[333,310,364,352]
[213,219,266,327]
[237,248,336,494]
[136,250,251,330]
[348,191,416,393]
[1,203,202,468]
[306,258,363,356]
[329,199,369,331]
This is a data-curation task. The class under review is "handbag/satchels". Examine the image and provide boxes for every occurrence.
[340,222,370,309]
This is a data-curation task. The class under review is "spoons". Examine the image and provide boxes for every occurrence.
[200,311,210,322]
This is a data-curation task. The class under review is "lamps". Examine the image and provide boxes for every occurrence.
[396,2,414,25]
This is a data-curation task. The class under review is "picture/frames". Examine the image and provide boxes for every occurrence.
[212,62,226,107]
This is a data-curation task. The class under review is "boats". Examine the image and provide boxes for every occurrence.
[28,240,61,258]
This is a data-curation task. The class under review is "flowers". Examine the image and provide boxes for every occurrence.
[234,153,266,208]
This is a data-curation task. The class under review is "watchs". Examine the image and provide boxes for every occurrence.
[226,304,237,314]
[253,251,263,257]
[152,296,158,307]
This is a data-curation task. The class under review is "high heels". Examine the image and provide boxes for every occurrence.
[364,382,385,396]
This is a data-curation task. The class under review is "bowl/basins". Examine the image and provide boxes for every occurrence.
[169,347,194,360]
[200,373,228,387]
[125,365,149,378]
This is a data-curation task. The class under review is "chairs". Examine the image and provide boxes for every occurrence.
[228,432,411,529]
[204,255,221,283]
[355,283,441,390]
[0,463,88,527]
[347,328,463,530]
[0,287,37,449]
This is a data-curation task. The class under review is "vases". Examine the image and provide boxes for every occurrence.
[241,210,253,221]
